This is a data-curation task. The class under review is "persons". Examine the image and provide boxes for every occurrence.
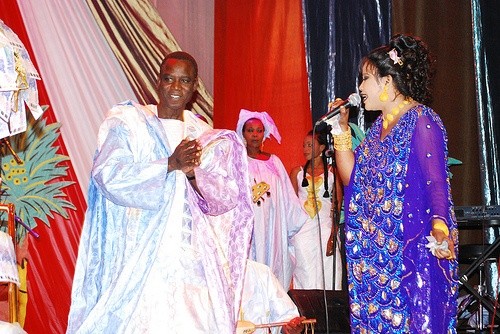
[290,129,343,334]
[67,51,253,334]
[235,259,308,334]
[235,109,307,293]
[327,33,458,334]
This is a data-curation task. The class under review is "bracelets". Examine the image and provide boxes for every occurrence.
[430,223,449,237]
[186,174,195,181]
[332,126,352,151]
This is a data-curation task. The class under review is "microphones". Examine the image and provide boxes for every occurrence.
[314,93,362,126]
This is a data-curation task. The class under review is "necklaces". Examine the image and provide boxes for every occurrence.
[383,97,413,130]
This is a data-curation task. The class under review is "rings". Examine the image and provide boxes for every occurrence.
[328,101,334,108]
[446,250,453,260]
[335,98,342,102]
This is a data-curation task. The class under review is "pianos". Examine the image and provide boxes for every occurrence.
[453,205,500,334]
